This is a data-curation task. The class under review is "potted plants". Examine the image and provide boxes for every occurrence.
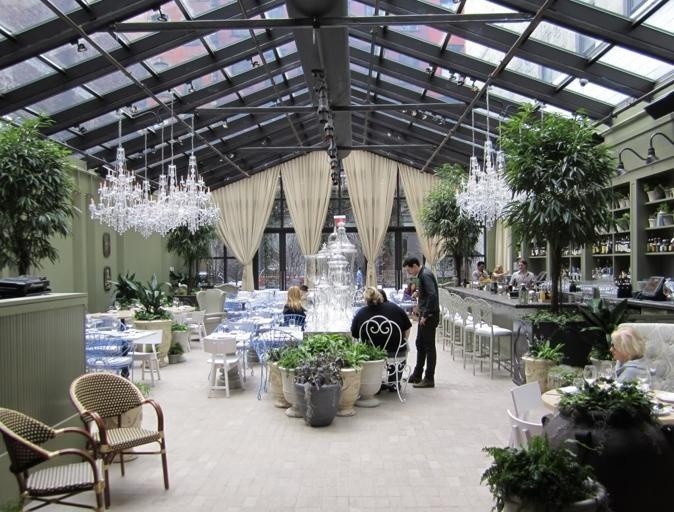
[579,298,627,371]
[521,335,565,395]
[542,371,674,510]
[495,102,620,367]
[267,333,387,428]
[480,432,609,512]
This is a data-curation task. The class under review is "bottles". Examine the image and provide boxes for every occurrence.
[647,233,674,252]
[591,235,630,254]
[618,275,632,298]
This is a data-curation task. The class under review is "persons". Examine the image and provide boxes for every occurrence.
[401,282,420,321]
[299,274,307,290]
[402,257,440,387]
[604,322,654,387]
[356,266,365,290]
[490,264,509,291]
[507,259,537,293]
[471,261,489,291]
[280,286,307,331]
[350,286,412,395]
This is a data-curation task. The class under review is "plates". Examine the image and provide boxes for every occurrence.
[656,391,674,403]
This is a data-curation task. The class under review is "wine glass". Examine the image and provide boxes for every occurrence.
[573,362,657,394]
[289,319,295,332]
[108,297,183,333]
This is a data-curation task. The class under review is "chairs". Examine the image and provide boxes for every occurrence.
[0,407,109,511]
[506,408,542,450]
[617,323,674,395]
[511,381,542,419]
[69,372,169,507]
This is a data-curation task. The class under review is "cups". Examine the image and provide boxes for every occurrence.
[218,324,223,336]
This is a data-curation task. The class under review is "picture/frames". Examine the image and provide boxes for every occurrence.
[104,266,112,291]
[102,232,110,256]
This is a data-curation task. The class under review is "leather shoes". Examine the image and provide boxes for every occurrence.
[413,379,434,387]
[403,375,422,383]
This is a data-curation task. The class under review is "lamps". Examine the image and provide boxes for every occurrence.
[454,85,536,228]
[89,91,220,240]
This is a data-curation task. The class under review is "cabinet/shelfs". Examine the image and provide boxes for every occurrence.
[633,155,674,302]
[548,242,582,280]
[582,169,632,298]
[523,239,548,275]
